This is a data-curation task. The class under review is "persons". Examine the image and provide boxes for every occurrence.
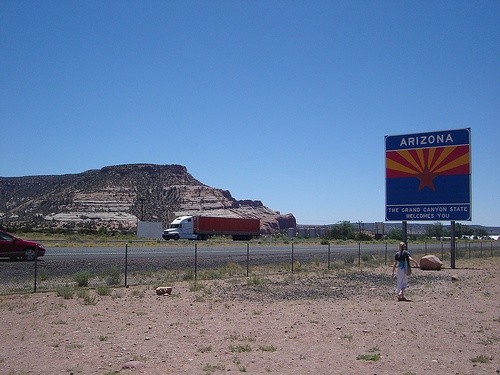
[392,242,421,301]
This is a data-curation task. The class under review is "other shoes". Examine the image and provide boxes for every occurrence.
[397,293,407,301]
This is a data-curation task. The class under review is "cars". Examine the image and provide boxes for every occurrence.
[1,229,45,261]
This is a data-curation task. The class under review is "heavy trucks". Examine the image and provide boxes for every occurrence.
[161,214,261,241]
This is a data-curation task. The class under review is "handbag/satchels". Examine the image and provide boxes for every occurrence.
[404,251,411,276]
